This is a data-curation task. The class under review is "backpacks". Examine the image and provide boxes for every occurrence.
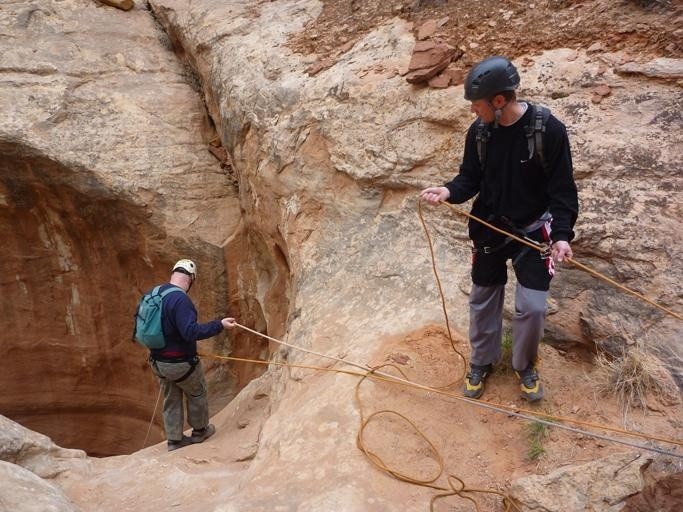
[134,295,166,350]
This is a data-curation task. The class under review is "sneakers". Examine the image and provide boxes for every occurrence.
[167,424,215,451]
[514,368,544,401]
[464,364,491,400]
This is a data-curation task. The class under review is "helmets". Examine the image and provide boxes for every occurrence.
[464,57,519,101]
[172,259,197,281]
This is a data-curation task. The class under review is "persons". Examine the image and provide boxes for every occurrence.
[142,257,237,452]
[419,55,578,402]
